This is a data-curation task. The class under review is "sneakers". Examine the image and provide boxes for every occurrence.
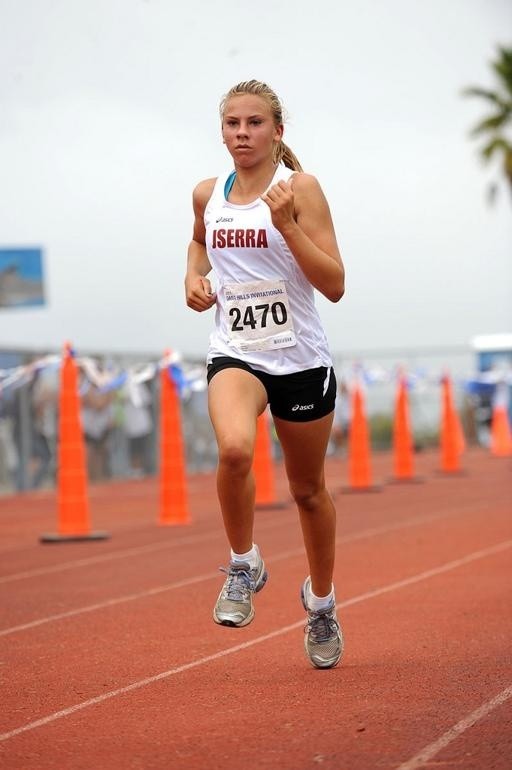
[213,557,267,628]
[302,576,342,667]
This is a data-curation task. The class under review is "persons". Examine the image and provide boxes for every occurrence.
[184,78,347,671]
